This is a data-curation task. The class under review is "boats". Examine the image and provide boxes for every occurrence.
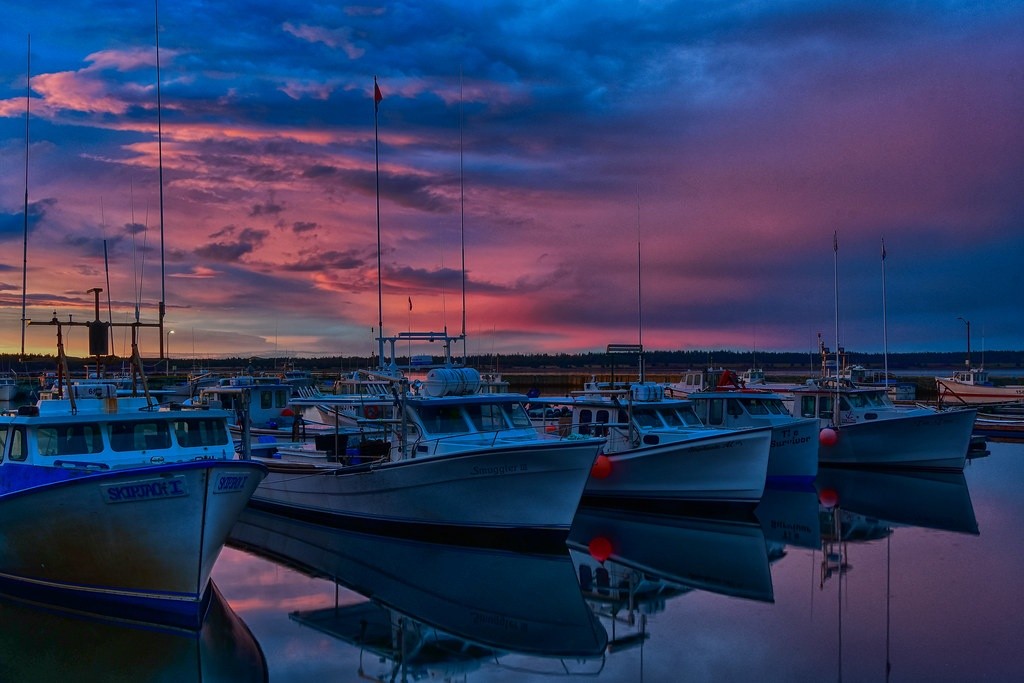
[0,577,270,683]
[814,464,980,536]
[678,369,822,490]
[225,507,650,683]
[753,488,823,551]
[0,82,1024,435]
[176,73,612,542]
[529,392,772,512]
[563,496,781,605]
[778,227,980,474]
[0,6,270,616]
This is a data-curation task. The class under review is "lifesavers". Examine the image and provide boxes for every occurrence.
[364,406,378,419]
